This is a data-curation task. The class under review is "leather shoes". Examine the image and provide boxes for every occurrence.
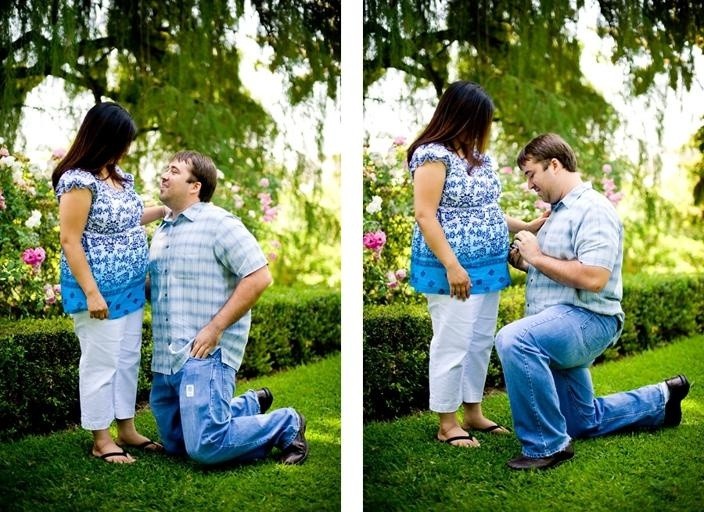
[505,442,576,473]
[664,374,690,427]
[278,411,309,468]
[255,387,273,414]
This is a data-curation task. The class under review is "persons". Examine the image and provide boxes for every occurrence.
[407,80,551,448]
[495,132,689,472]
[52,102,172,464]
[148,149,309,464]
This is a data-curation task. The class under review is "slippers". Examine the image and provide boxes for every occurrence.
[116,435,165,451]
[436,426,481,448]
[91,446,136,464]
[461,415,514,436]
